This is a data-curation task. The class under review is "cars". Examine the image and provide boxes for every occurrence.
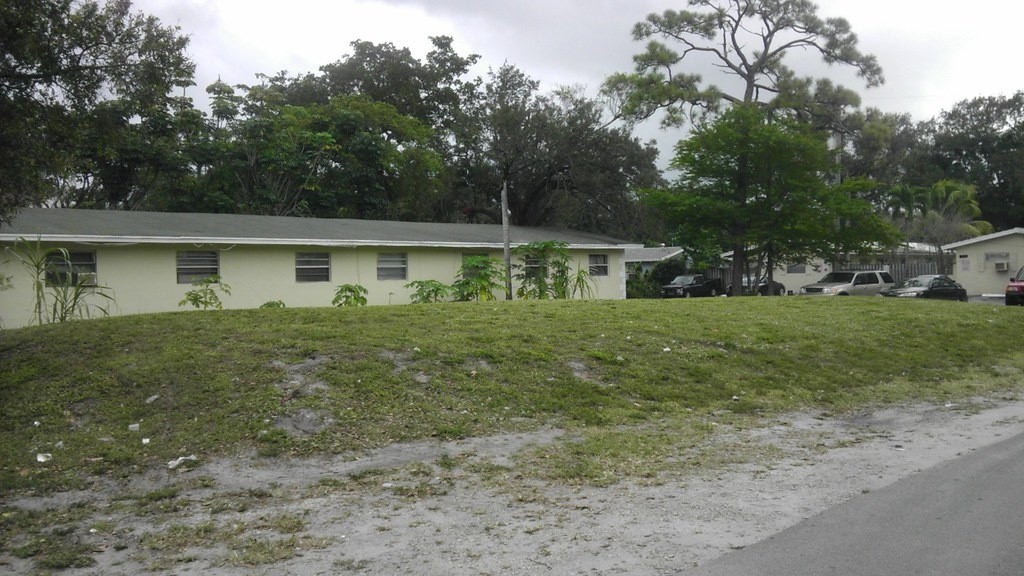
[725,276,786,297]
[1005,265,1024,306]
[875,274,969,304]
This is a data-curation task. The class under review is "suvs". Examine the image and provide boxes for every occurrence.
[799,270,896,299]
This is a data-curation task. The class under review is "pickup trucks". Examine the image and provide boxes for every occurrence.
[661,274,721,298]
